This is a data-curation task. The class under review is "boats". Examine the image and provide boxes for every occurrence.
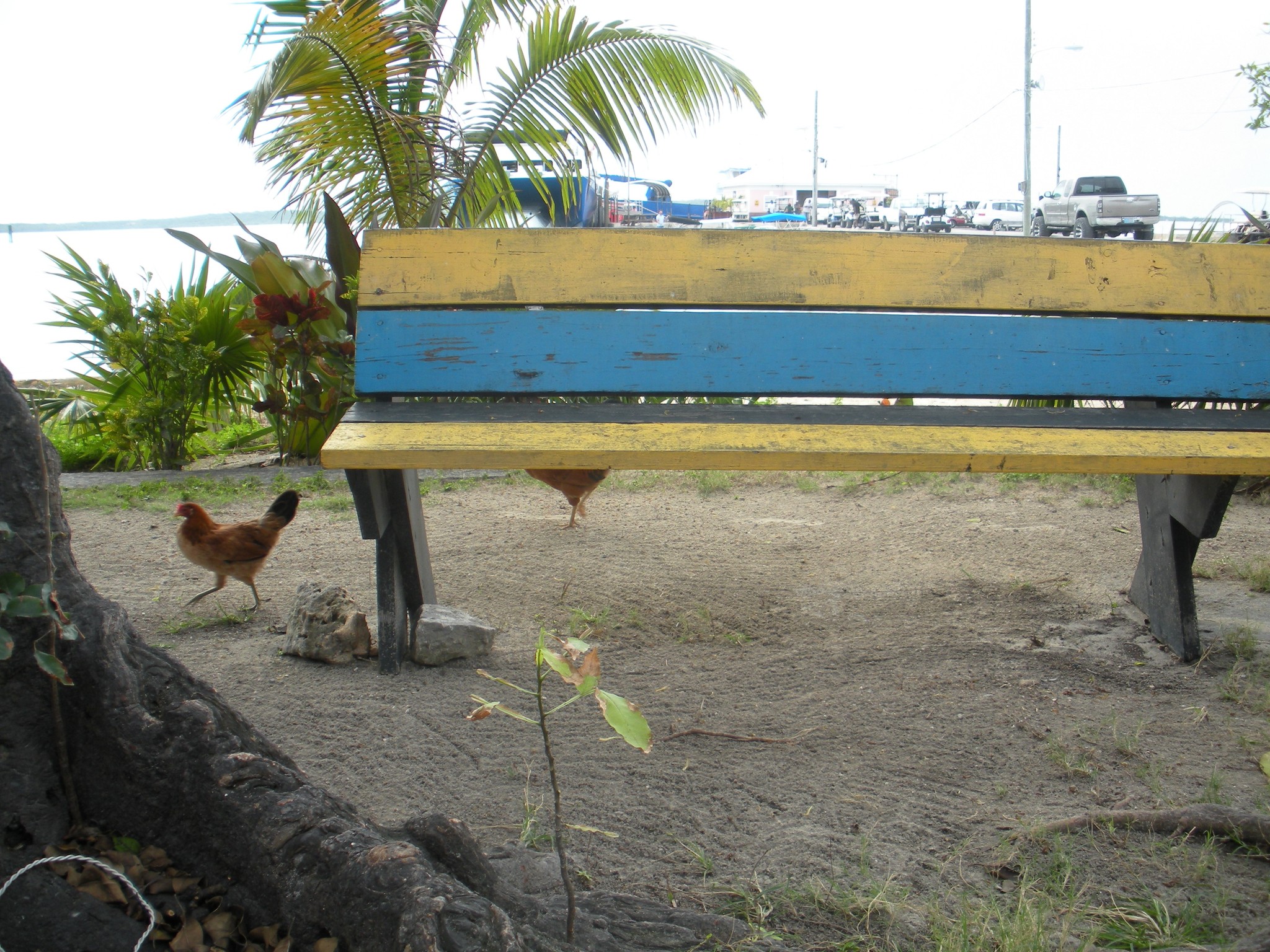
[745,213,809,230]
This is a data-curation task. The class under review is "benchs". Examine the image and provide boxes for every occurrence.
[321,229,1270,669]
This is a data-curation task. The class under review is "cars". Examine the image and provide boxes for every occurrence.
[946,211,976,228]
[972,200,1035,231]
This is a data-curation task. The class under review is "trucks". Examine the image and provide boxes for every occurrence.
[829,194,878,229]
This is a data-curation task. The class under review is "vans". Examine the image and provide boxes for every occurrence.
[802,196,837,225]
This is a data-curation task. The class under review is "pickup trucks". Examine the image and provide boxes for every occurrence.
[1032,176,1161,241]
[878,196,929,232]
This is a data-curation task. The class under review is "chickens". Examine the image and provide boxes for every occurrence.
[524,468,612,530]
[173,489,301,615]
